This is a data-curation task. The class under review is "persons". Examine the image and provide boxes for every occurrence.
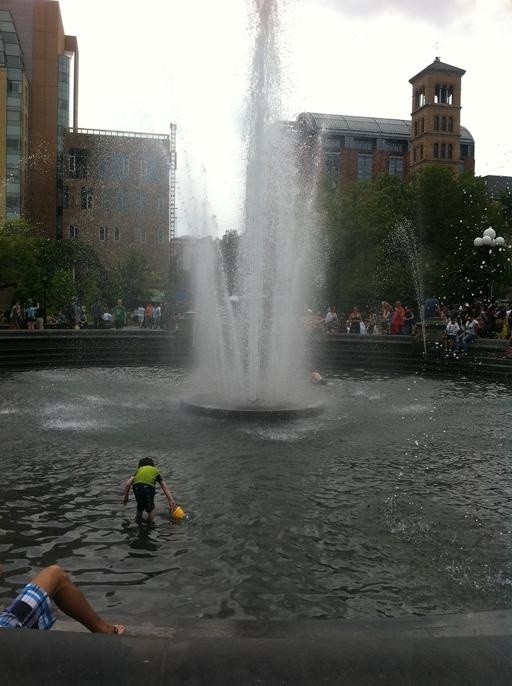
[302,301,414,335]
[1,563,127,636]
[3,298,39,330]
[67,296,177,330]
[122,456,175,521]
[436,299,512,352]
[309,366,328,386]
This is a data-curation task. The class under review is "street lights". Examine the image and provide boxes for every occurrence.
[468,225,506,311]
[39,268,53,328]
[230,282,238,312]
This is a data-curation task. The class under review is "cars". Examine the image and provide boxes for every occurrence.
[129,306,162,325]
